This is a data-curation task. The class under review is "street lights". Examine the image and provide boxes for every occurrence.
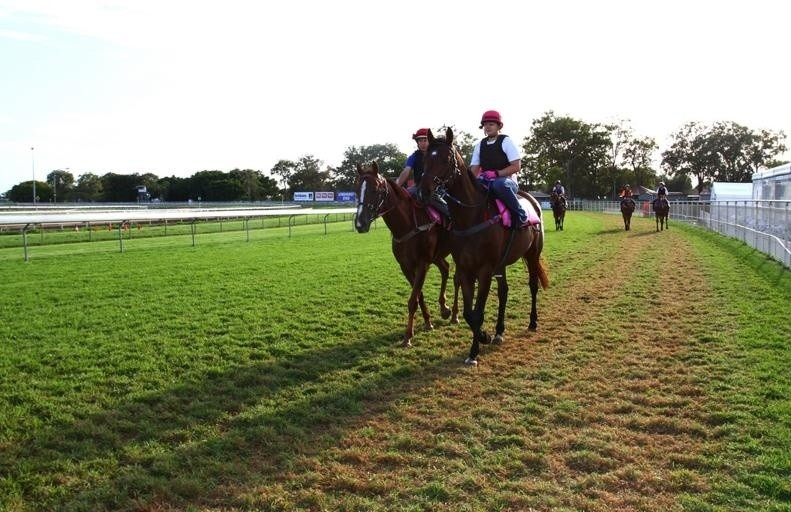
[31,147,36,204]
[596,191,607,201]
[53,167,70,205]
[278,193,283,204]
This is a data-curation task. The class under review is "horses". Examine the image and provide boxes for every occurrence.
[550,192,567,231]
[654,195,670,231]
[354,159,462,349]
[620,198,635,231]
[415,127,550,368]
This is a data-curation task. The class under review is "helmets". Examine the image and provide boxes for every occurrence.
[481,111,503,129]
[415,129,429,142]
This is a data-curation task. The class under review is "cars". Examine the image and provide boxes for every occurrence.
[151,197,161,203]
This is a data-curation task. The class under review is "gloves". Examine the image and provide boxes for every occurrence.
[480,170,497,179]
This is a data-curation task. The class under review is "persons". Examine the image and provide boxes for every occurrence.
[620,184,636,211]
[397,128,453,229]
[550,180,568,208]
[469,111,531,222]
[653,182,671,211]
